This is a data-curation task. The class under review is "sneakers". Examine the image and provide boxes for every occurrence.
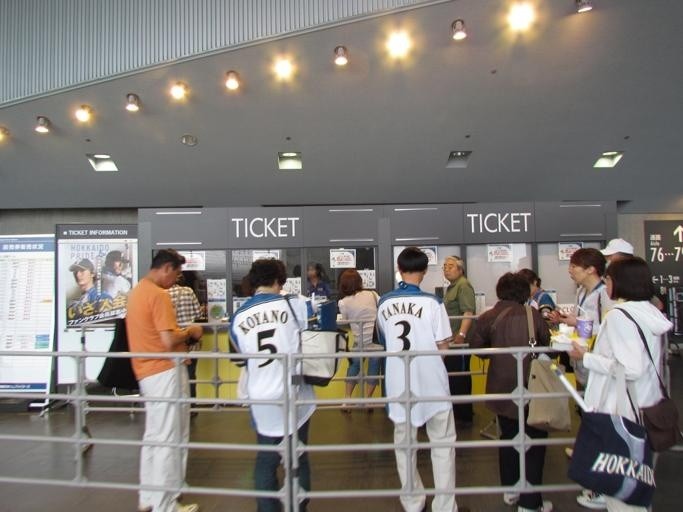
[172,501,199,512]
[517,501,552,512]
[502,493,520,505]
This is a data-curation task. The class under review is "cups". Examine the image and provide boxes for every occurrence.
[575,316,593,338]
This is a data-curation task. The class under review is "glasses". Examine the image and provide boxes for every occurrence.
[441,266,452,270]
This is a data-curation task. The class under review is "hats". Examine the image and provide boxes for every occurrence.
[104,250,128,263]
[599,239,633,255]
[68,259,94,271]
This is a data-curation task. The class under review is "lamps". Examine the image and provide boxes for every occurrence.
[226,69,240,90]
[34,116,50,132]
[170,82,186,100]
[574,0,593,15]
[451,20,467,42]
[333,47,347,66]
[76,103,93,123]
[125,93,140,112]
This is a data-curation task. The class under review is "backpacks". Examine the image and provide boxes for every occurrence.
[567,413,656,506]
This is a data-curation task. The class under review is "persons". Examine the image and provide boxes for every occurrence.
[517,268,555,321]
[569,256,674,512]
[375,247,459,511]
[164,273,204,418]
[304,262,331,301]
[66,258,113,324]
[599,238,664,312]
[441,256,476,418]
[121,248,203,512]
[337,269,382,413]
[227,258,318,511]
[546,248,614,458]
[469,272,553,511]
[100,250,130,298]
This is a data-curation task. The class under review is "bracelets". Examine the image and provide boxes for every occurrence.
[458,333,465,338]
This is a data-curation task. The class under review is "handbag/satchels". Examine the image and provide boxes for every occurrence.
[291,331,348,386]
[526,359,571,432]
[641,398,682,451]
